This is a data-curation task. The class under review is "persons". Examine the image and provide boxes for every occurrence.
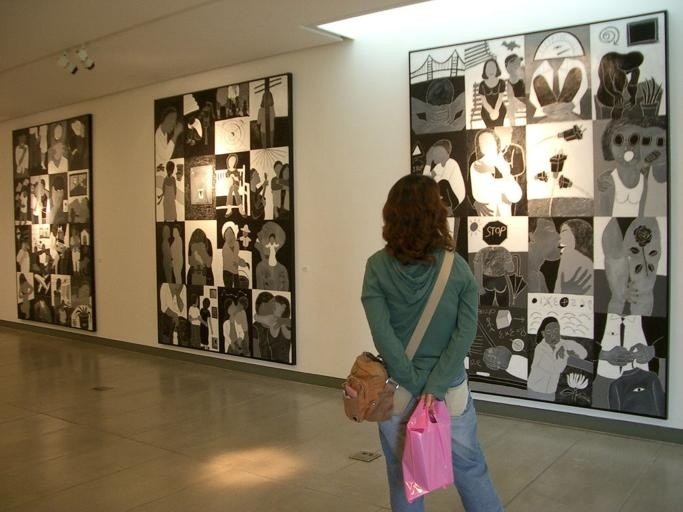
[359,173,504,511]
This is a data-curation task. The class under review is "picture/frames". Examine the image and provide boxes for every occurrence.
[10,113,96,334]
[150,73,297,368]
[407,10,671,421]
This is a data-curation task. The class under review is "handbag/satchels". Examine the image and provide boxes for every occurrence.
[400,391,455,503]
[340,350,396,423]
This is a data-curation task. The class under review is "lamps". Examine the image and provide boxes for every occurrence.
[56,43,95,75]
